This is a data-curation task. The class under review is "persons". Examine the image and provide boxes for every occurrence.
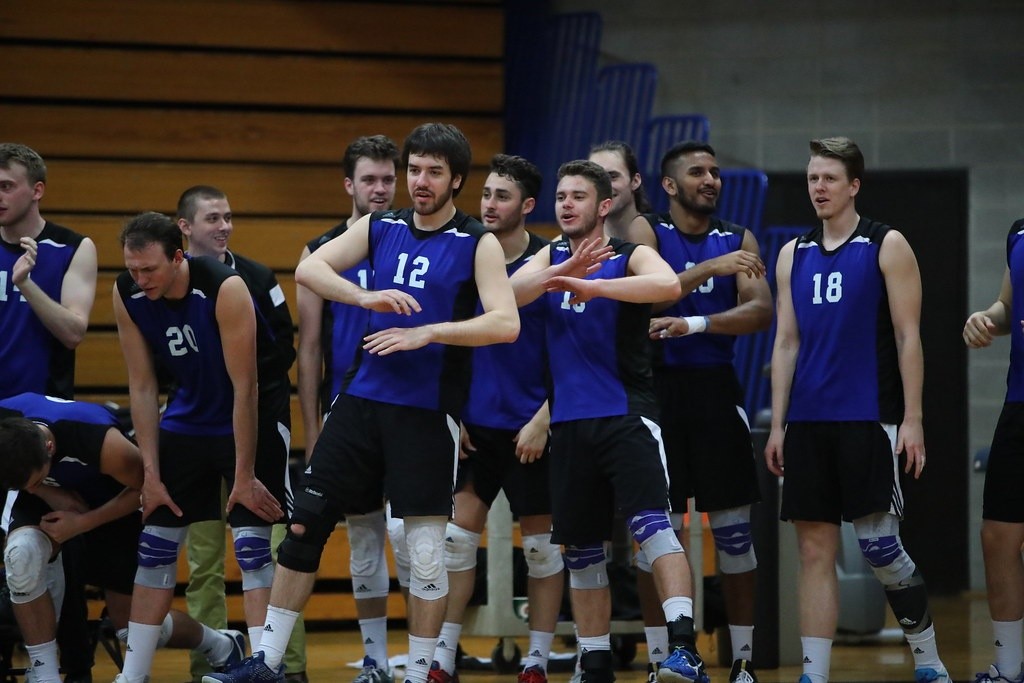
[174,133,642,683]
[765,134,954,683]
[0,390,247,683]
[112,213,285,683]
[963,217,1024,683]
[627,139,774,683]
[294,118,522,683]
[508,159,712,683]
[0,143,98,683]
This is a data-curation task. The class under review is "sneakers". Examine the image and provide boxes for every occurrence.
[202,649,288,683]
[209,628,246,672]
[351,654,394,683]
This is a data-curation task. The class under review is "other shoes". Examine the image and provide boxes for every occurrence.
[973,664,1024,683]
[579,649,616,683]
[25,667,61,683]
[729,658,758,683]
[914,667,952,683]
[426,667,459,683]
[656,642,710,683]
[516,664,547,683]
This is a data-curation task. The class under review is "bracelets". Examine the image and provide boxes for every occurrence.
[703,315,710,332]
